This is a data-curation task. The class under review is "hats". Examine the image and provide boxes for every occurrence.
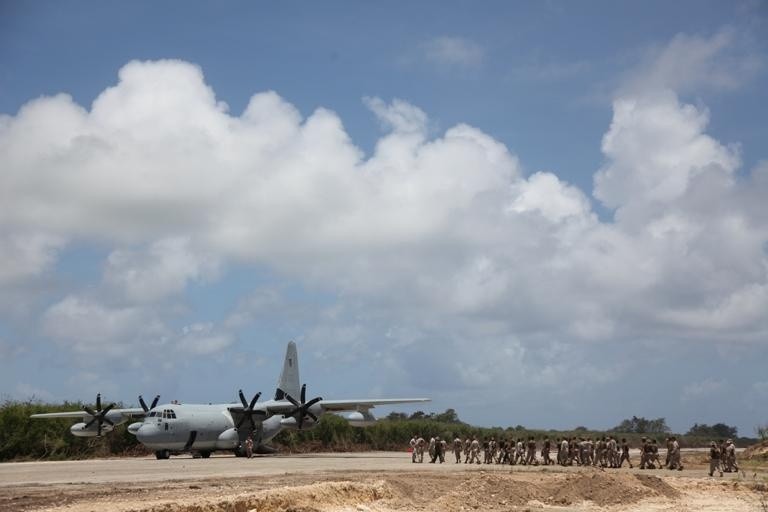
[711,441,716,446]
[727,439,732,443]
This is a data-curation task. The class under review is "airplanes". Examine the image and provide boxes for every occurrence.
[30,341,430,459]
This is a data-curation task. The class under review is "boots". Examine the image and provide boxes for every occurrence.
[708,472,723,477]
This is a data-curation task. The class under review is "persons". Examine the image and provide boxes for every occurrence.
[452,434,684,471]
[707,439,740,477]
[409,433,447,464]
[245,436,254,459]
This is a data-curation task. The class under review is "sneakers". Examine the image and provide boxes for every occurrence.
[670,466,683,471]
[577,463,595,466]
[723,468,738,472]
[630,464,633,468]
[456,460,555,465]
[638,464,662,469]
[412,460,445,463]
[600,465,621,468]
